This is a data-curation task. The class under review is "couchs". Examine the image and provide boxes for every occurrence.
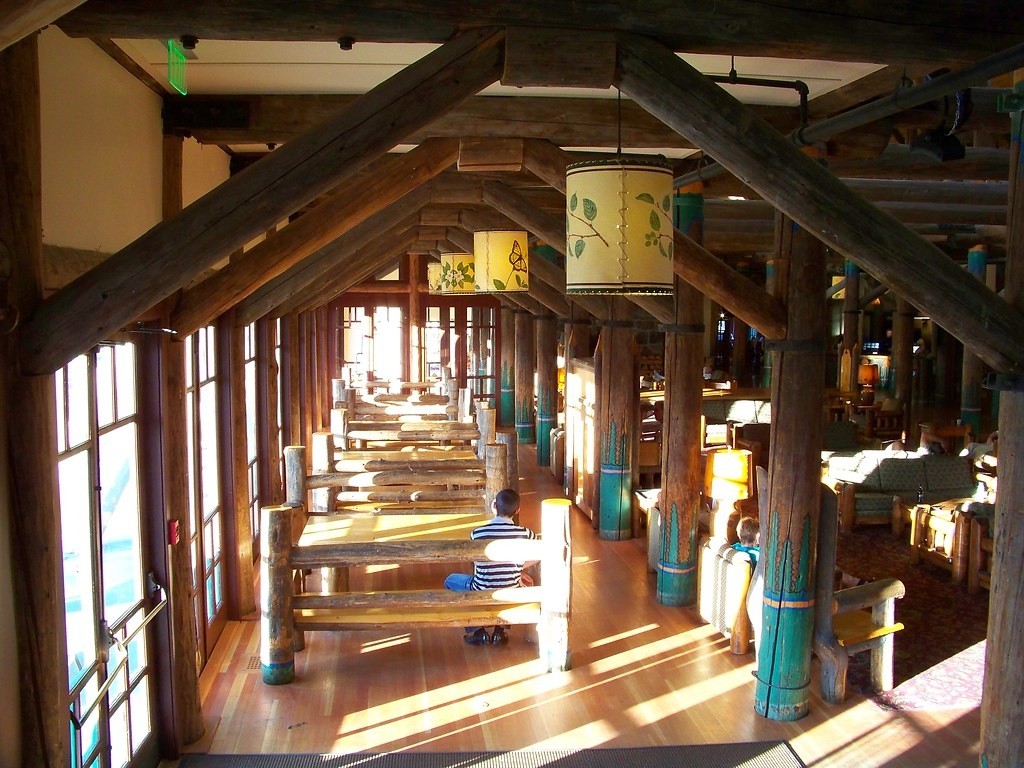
[697,396,995,595]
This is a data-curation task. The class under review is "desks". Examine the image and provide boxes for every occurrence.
[297,514,495,541]
[335,449,477,460]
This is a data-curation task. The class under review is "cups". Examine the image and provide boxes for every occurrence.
[957,420,961,427]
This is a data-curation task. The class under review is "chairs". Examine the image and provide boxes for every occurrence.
[633,402,902,706]
[826,392,974,451]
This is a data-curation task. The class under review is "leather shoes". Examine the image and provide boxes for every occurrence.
[491,632,507,645]
[464,633,489,646]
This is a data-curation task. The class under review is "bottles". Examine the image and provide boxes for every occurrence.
[861,384,874,406]
[917,483,924,504]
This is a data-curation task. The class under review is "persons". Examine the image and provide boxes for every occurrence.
[749,337,765,388]
[881,436,998,504]
[878,328,892,354]
[444,489,542,645]
[731,517,878,587]
[642,365,714,383]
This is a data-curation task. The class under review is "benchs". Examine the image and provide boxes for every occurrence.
[258,369,572,687]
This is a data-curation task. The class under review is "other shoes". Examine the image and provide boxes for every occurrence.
[856,578,875,587]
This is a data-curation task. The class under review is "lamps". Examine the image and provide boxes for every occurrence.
[564,90,674,297]
[859,364,878,405]
[427,262,441,296]
[473,208,529,293]
[441,252,473,296]
